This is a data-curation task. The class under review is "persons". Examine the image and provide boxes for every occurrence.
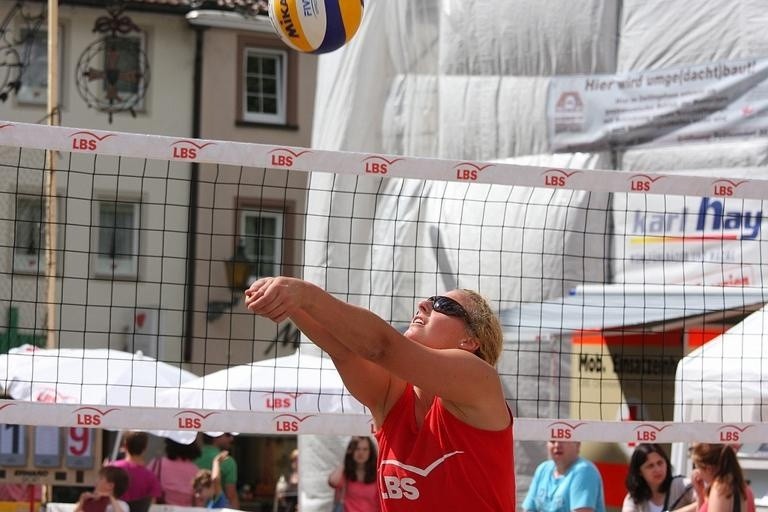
[272,447,298,512]
[191,430,245,511]
[689,442,757,512]
[145,437,203,507]
[621,442,701,511]
[72,465,130,511]
[520,440,607,511]
[245,275,516,512]
[192,449,230,509]
[109,431,162,511]
[328,435,380,511]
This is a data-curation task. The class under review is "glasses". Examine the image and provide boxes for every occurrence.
[428,295,480,339]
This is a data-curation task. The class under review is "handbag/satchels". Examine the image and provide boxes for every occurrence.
[332,481,346,512]
[152,458,166,505]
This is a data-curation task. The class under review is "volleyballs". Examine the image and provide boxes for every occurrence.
[266,0,366,55]
